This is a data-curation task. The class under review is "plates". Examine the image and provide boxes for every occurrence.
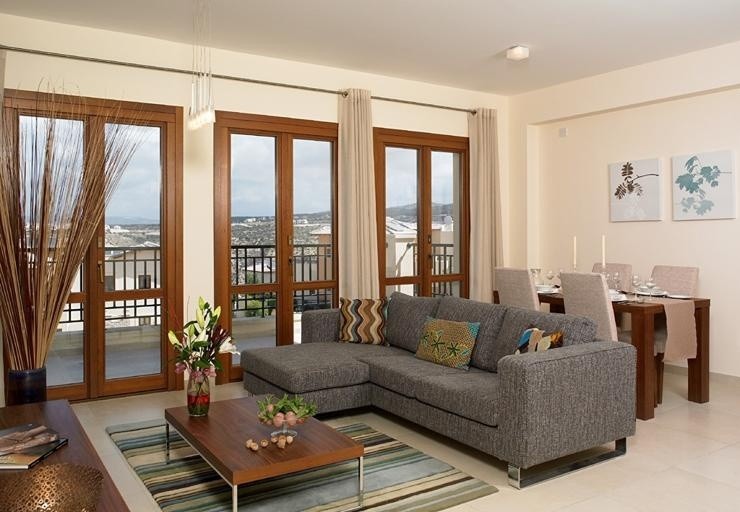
[635,291,667,296]
[668,294,693,299]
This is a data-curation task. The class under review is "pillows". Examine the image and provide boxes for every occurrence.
[339,289,599,370]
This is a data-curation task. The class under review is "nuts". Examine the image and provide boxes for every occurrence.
[245,435,293,450]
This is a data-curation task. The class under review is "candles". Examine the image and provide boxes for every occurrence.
[573,236,576,267]
[602,235,606,268]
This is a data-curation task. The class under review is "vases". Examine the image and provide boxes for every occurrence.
[186,371,210,418]
[6,367,46,403]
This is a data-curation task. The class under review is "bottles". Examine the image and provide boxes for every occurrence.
[530,269,544,287]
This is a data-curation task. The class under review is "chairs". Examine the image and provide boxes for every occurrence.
[561,271,619,342]
[494,266,541,311]
[592,262,632,293]
[617,264,700,405]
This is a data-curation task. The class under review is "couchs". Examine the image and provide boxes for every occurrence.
[241,309,638,492]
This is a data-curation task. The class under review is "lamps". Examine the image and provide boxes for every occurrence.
[185,0,216,130]
[507,45,530,62]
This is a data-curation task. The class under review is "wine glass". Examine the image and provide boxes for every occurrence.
[255,410,306,440]
[546,271,555,289]
[604,270,656,304]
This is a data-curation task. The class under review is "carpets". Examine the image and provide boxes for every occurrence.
[104,417,500,511]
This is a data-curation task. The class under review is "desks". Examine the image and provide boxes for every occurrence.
[0,398,129,511]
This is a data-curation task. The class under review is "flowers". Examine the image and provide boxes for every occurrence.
[167,296,241,413]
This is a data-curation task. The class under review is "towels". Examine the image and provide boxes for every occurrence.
[626,294,698,363]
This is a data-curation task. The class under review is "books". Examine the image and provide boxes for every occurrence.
[1,423,68,470]
